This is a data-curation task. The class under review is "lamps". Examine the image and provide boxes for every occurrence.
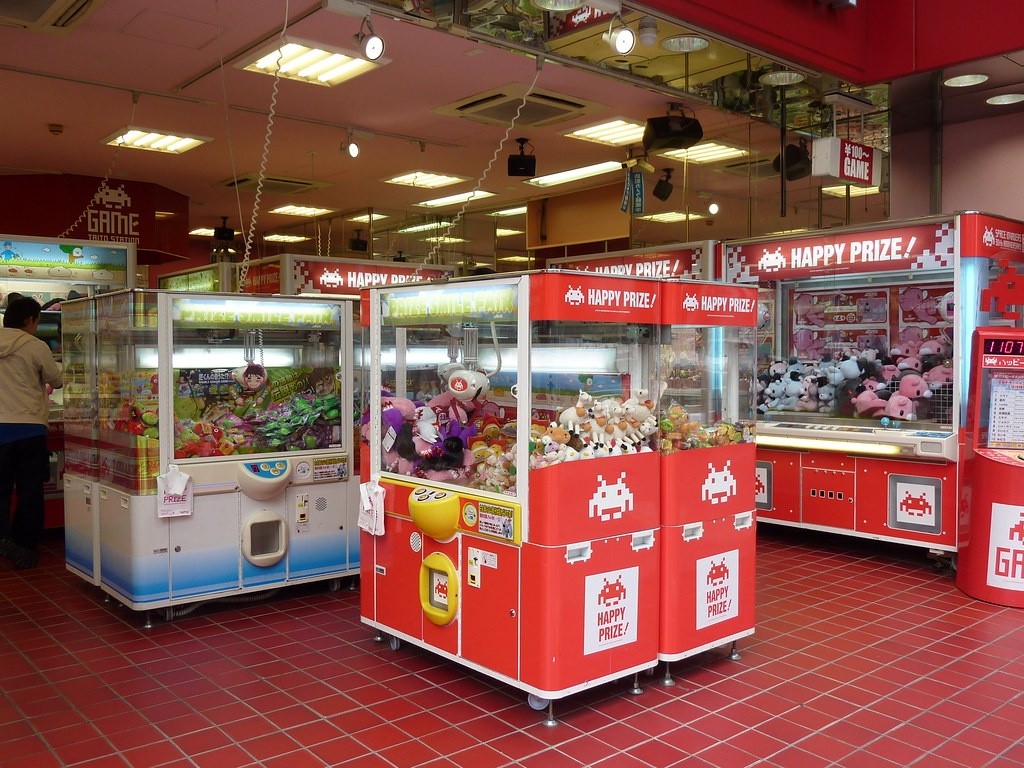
[352,33,385,62]
[393,251,409,263]
[349,229,367,252]
[602,26,635,56]
[508,154,536,176]
[213,216,234,241]
[642,116,703,152]
[707,203,721,216]
[341,142,360,159]
[772,144,812,181]
[638,18,657,45]
[653,168,675,201]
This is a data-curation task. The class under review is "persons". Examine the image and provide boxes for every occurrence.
[0,292,63,570]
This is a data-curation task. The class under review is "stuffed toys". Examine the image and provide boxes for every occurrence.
[116,404,237,459]
[361,386,756,501]
[746,337,954,422]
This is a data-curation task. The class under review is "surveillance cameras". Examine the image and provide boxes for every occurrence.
[638,17,657,45]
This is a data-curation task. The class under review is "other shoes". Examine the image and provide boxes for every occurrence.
[0,537,39,569]
